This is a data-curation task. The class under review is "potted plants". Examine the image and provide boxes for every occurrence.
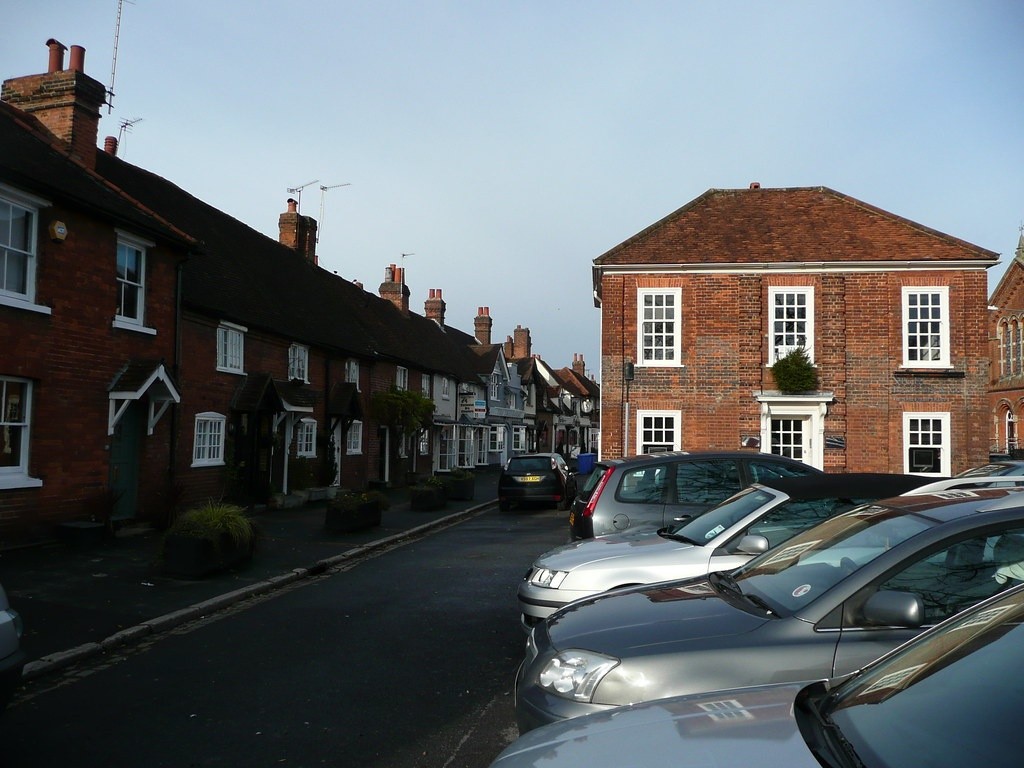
[325,491,382,531]
[154,495,259,575]
[447,464,475,500]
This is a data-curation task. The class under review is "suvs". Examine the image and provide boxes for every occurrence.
[513,475,1024,736]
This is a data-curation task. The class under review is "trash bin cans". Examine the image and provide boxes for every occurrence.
[577,453,596,475]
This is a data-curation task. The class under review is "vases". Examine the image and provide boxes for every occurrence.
[411,486,443,510]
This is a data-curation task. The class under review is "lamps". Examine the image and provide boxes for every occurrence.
[228,421,235,437]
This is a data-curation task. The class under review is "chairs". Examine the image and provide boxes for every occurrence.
[982,534,1024,599]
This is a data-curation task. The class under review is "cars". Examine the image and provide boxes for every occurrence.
[569,451,825,542]
[517,472,978,633]
[485,583,1024,768]
[955,459,1023,487]
[499,452,578,510]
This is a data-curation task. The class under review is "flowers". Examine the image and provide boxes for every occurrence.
[415,475,446,492]
